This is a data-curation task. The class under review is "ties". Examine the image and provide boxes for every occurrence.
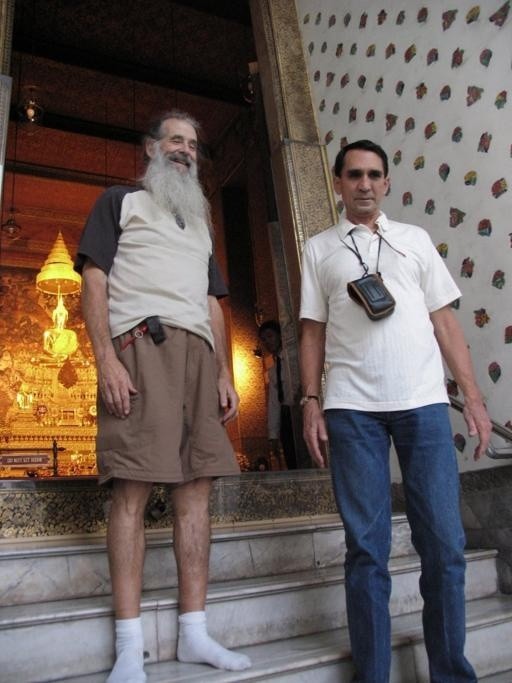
[276,356,284,402]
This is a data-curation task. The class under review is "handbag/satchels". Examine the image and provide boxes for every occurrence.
[347,274,395,320]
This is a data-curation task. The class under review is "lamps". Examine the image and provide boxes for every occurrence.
[11,82,48,134]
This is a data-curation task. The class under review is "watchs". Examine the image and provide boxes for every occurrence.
[299,394,319,408]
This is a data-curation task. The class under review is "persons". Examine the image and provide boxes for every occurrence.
[259,320,298,470]
[297,140,494,682]
[74,107,252,682]
